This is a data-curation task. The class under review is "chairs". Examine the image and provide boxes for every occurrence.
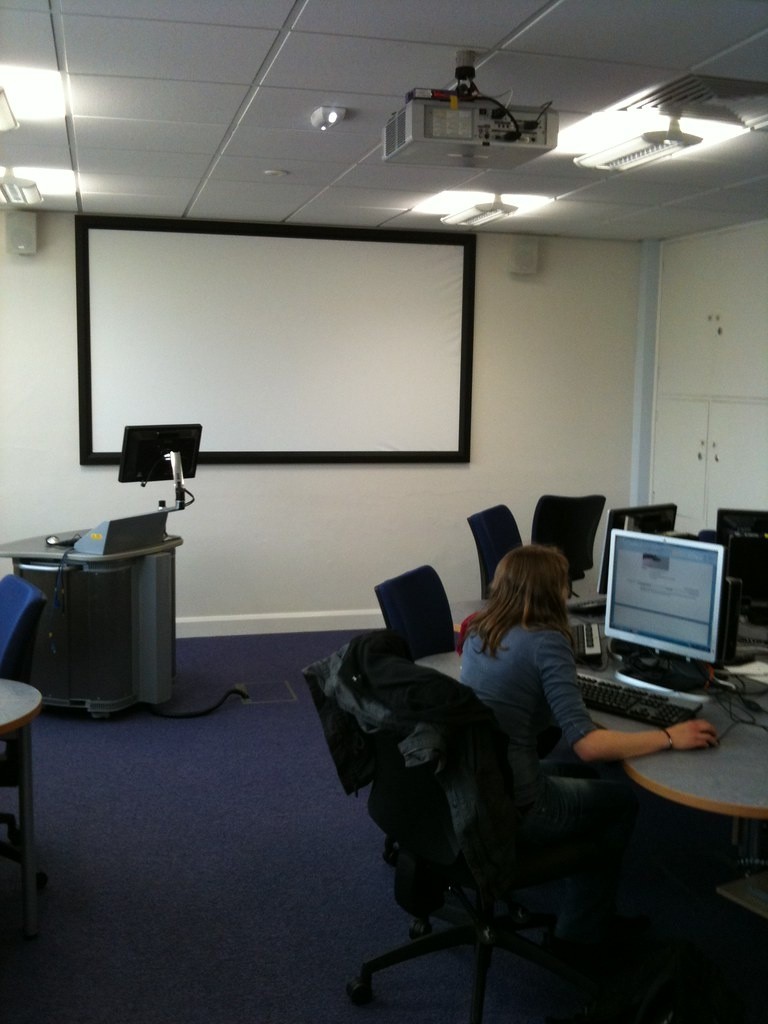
[0,574,49,890]
[469,503,523,601]
[531,494,608,598]
[376,564,455,666]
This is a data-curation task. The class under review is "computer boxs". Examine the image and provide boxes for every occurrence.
[716,575,742,662]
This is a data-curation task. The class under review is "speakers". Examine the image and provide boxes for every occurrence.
[509,235,539,275]
[6,210,38,255]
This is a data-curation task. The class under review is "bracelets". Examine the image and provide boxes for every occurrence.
[661,729,672,751]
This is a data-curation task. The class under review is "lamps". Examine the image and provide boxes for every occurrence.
[1,167,45,206]
[438,193,517,228]
[0,86,20,134]
[574,117,703,172]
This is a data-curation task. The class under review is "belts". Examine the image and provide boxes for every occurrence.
[514,795,539,813]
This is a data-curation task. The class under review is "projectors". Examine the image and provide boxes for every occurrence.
[384,100,559,169]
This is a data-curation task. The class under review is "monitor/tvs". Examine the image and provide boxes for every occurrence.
[119,424,202,481]
[596,503,768,693]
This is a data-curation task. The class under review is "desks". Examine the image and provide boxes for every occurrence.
[552,600,767,867]
[0,678,43,940]
[300,627,590,1024]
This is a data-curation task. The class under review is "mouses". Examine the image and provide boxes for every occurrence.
[46,535,60,546]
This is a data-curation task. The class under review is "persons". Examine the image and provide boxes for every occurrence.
[458,544,717,941]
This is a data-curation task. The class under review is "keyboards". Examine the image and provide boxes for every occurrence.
[568,597,608,614]
[57,538,80,549]
[577,672,703,728]
[571,622,602,664]
